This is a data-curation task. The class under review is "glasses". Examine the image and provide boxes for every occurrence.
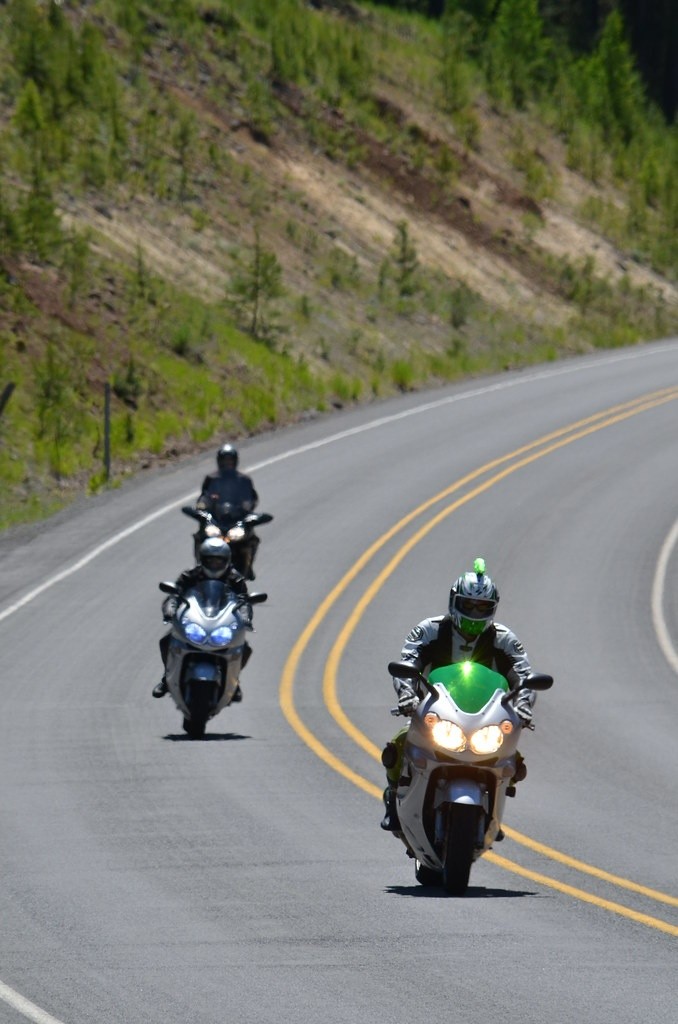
[458,599,492,612]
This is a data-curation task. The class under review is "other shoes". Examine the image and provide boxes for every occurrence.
[152,682,169,698]
[240,564,255,580]
[231,687,241,701]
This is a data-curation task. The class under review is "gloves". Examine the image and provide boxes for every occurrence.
[512,700,533,728]
[163,607,176,618]
[240,613,251,625]
[397,692,420,717]
[196,502,206,510]
[243,501,252,511]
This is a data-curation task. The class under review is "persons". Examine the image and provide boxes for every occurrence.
[188,445,258,581]
[379,557,539,839]
[152,538,253,703]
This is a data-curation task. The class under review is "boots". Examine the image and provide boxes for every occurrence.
[496,830,504,841]
[380,792,399,830]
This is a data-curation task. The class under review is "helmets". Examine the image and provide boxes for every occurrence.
[449,557,500,636]
[217,443,238,471]
[200,536,232,578]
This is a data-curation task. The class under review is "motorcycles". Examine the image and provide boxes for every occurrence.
[179,503,274,592]
[382,660,555,894]
[154,580,266,735]
